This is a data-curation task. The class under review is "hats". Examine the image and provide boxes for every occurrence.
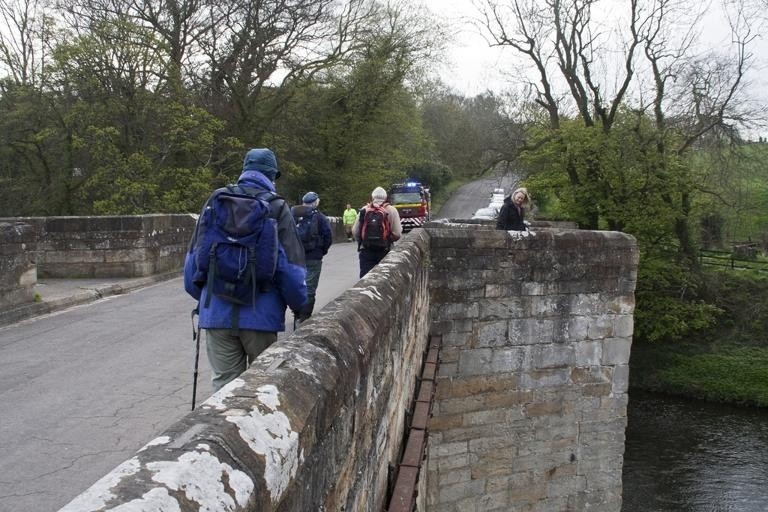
[372,187,387,201]
[302,191,319,203]
[243,149,278,181]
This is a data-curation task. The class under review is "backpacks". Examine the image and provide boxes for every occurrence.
[193,184,285,305]
[290,206,319,251]
[360,203,391,249]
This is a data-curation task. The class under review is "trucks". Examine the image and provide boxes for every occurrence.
[386,183,431,232]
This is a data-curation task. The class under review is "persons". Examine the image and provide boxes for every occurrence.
[291,192,332,329]
[183,149,308,392]
[497,187,530,229]
[343,203,357,242]
[352,185,402,276]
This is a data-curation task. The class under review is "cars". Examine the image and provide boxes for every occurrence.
[471,208,499,222]
[488,188,508,214]
